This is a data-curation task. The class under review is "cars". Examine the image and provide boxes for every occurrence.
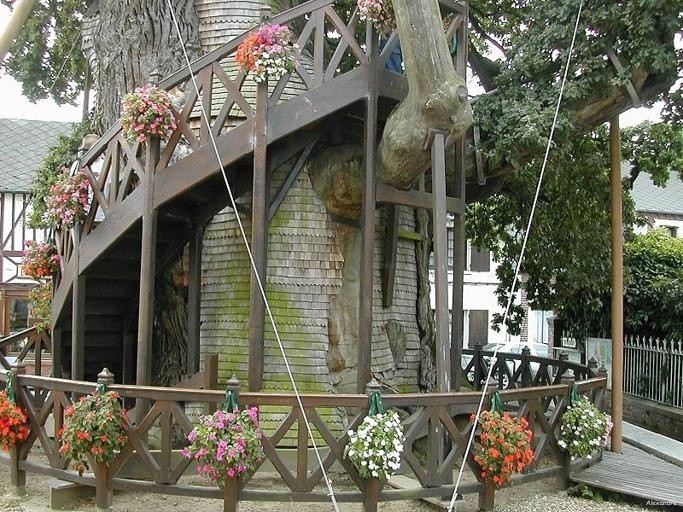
[457,342,550,389]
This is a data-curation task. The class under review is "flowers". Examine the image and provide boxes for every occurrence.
[357,0,397,35]
[0,388,31,452]
[20,240,60,279]
[556,394,614,463]
[342,409,406,480]
[182,410,266,487]
[117,86,180,145]
[234,15,301,84]
[27,281,55,333]
[469,410,536,489]
[56,385,130,478]
[40,168,92,230]
